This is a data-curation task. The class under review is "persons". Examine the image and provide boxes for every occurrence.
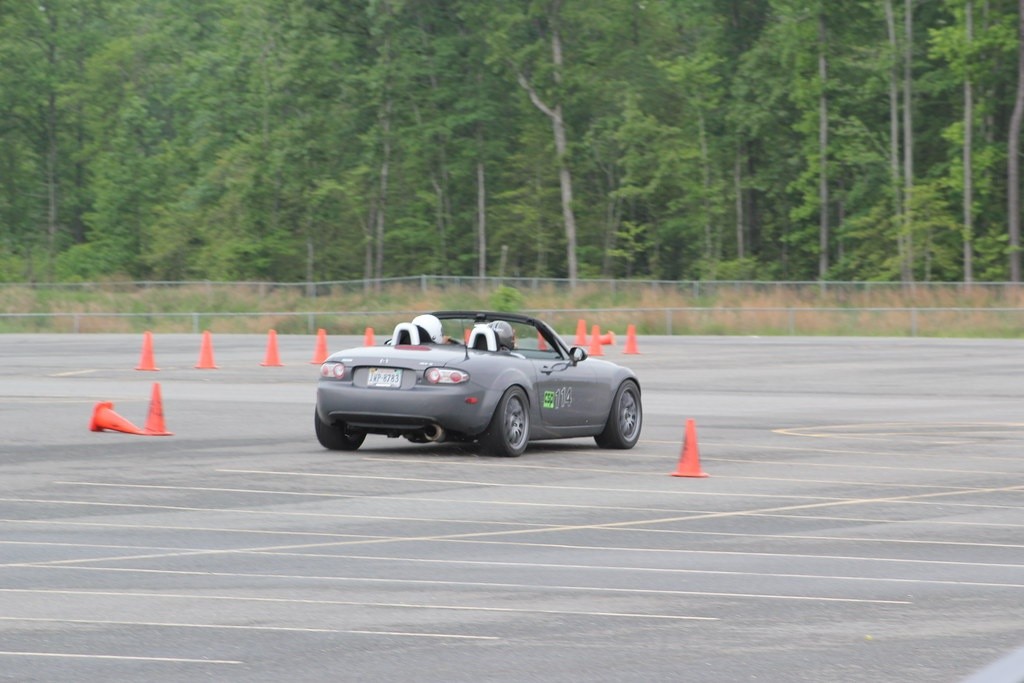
[487,320,515,351]
[412,314,452,346]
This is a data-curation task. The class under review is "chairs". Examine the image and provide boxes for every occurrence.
[467,327,500,352]
[392,322,433,345]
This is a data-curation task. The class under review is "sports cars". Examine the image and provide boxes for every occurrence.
[314,312,643,456]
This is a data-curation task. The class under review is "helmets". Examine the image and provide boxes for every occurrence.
[488,320,514,350]
[412,314,442,343]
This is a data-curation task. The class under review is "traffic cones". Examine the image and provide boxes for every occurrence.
[308,328,331,364]
[537,330,548,351]
[259,329,286,366]
[573,319,615,355]
[622,324,641,355]
[89,382,174,436]
[464,328,472,345]
[670,418,708,477]
[363,327,377,346]
[134,331,160,371]
[193,330,220,369]
